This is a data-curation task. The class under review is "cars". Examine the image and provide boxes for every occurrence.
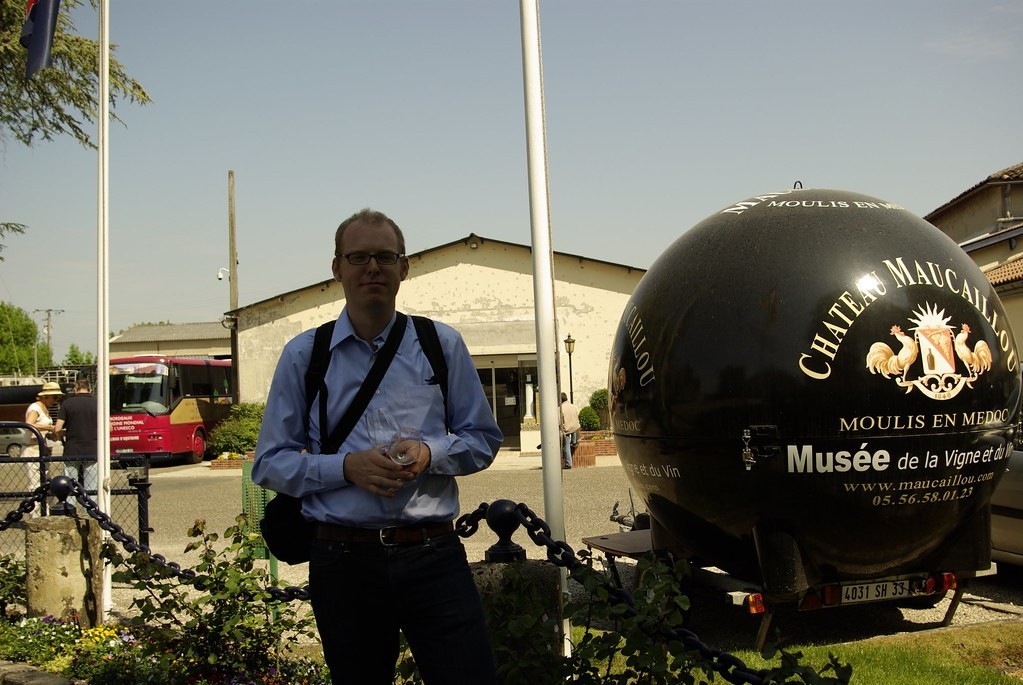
[0,420,39,457]
[989,411,1023,569]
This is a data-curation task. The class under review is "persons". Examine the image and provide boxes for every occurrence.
[250,209,504,685]
[558,392,581,469]
[20,382,63,518]
[54,380,97,512]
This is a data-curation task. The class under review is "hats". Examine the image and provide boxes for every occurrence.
[38,382,63,396]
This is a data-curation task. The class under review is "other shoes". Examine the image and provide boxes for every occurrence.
[573,442,579,447]
[562,465,571,469]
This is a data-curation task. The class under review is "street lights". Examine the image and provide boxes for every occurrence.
[564,333,575,405]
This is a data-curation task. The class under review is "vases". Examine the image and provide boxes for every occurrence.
[577,436,617,455]
[211,457,253,470]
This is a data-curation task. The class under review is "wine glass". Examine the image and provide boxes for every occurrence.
[388,428,422,466]
[366,406,418,491]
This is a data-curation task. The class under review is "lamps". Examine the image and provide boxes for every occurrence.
[470,242,478,249]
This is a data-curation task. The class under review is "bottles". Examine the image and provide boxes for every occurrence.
[927,348,935,371]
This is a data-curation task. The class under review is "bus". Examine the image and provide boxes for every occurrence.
[109,353,231,465]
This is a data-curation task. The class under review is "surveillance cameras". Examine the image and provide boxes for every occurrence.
[217,273,223,280]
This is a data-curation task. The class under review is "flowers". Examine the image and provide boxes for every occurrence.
[582,433,614,440]
[217,452,248,460]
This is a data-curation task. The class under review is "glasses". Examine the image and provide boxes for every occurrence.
[53,396,58,400]
[335,252,405,265]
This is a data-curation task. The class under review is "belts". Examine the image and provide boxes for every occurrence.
[316,522,453,544]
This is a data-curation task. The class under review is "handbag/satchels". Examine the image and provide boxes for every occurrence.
[259,492,314,565]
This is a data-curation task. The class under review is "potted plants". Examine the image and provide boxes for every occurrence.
[589,388,611,429]
